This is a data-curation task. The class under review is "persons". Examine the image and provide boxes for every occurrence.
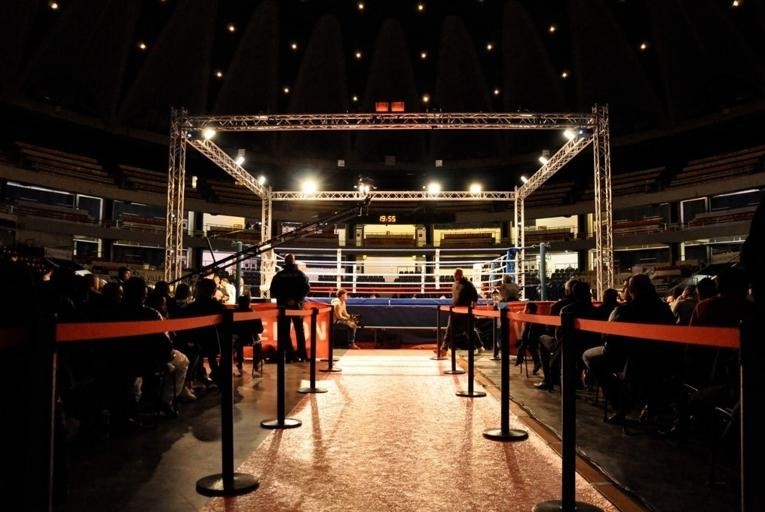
[440,269,485,356]
[1,250,264,509]
[493,269,763,458]
[737,185,765,374]
[270,255,309,363]
[331,289,359,349]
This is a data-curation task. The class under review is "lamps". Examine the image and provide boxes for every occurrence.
[374,99,405,114]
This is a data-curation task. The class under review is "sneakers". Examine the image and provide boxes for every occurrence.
[474,347,485,355]
[348,343,359,349]
[162,403,179,416]
[183,386,197,400]
[533,363,541,373]
[208,373,219,389]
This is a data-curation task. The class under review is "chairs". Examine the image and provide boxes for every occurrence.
[0,297,260,512]
[520,310,764,512]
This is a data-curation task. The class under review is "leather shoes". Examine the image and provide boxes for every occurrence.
[534,381,549,389]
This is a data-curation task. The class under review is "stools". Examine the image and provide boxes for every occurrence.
[334,325,349,349]
